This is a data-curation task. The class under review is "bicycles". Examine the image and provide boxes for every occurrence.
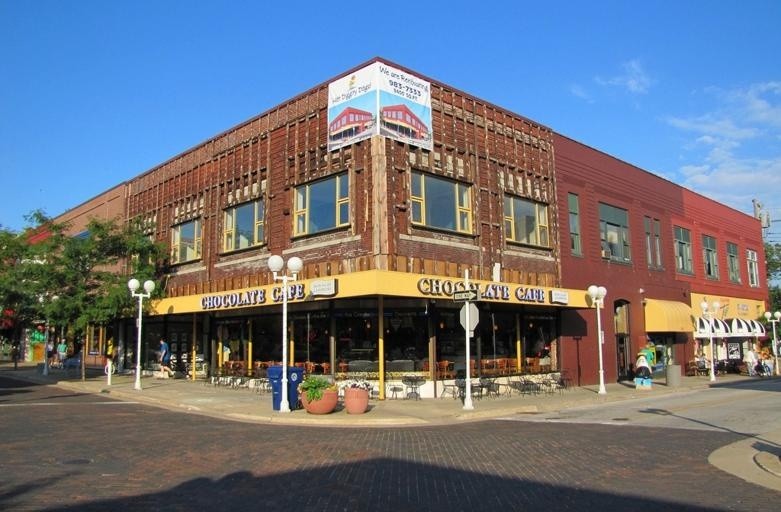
[754,364,771,377]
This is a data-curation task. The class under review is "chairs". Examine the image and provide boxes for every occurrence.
[688,360,708,376]
[204,358,348,392]
[390,372,572,401]
[419,354,546,379]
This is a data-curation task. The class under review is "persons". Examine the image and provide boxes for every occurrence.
[47,338,54,367]
[216,340,231,365]
[691,350,702,369]
[56,338,68,369]
[729,344,739,355]
[9,345,21,370]
[754,359,765,376]
[763,352,774,372]
[111,343,122,370]
[697,353,711,369]
[157,339,175,379]
[742,347,755,376]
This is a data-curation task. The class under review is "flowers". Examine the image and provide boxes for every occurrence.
[340,379,373,391]
[297,376,339,403]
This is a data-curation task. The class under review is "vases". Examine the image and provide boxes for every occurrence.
[301,390,338,413]
[345,388,369,414]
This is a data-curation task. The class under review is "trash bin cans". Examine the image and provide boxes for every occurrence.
[268,366,304,410]
[667,365,682,387]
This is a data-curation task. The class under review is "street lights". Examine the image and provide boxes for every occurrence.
[587,284,607,393]
[267,254,305,413]
[699,301,719,383]
[38,292,59,376]
[128,278,156,390]
[764,310,781,376]
